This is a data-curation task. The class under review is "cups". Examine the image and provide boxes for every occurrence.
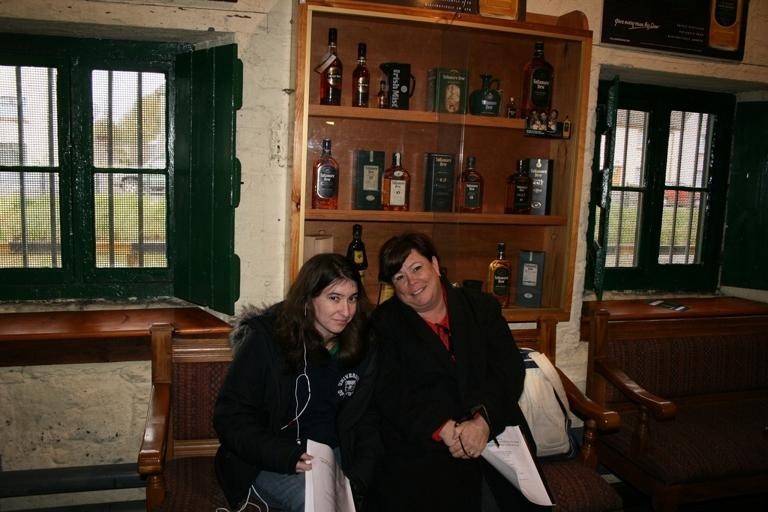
[462,278,483,294]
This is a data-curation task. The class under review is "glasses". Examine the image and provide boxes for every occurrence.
[453,404,500,458]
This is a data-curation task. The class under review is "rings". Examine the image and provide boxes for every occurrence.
[469,451,475,456]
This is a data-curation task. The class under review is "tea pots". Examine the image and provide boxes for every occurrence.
[380,62,415,109]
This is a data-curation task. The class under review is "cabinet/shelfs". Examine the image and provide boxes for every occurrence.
[280,3,597,325]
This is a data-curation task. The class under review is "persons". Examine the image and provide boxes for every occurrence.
[359,234,526,512]
[213,253,371,512]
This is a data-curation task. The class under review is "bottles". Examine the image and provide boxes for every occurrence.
[453,156,483,211]
[377,79,388,106]
[707,1,742,52]
[346,221,368,288]
[489,240,512,307]
[523,40,555,125]
[562,115,571,139]
[311,138,340,212]
[503,158,532,214]
[380,153,410,211]
[469,73,502,117]
[351,40,371,108]
[377,282,394,308]
[317,25,343,106]
[506,97,518,118]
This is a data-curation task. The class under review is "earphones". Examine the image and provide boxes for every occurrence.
[305,301,309,309]
[296,439,301,446]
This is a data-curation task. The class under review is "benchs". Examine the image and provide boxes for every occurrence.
[136,298,626,508]
[587,309,768,510]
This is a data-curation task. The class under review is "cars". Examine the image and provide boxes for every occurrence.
[663,181,698,206]
[113,157,166,194]
